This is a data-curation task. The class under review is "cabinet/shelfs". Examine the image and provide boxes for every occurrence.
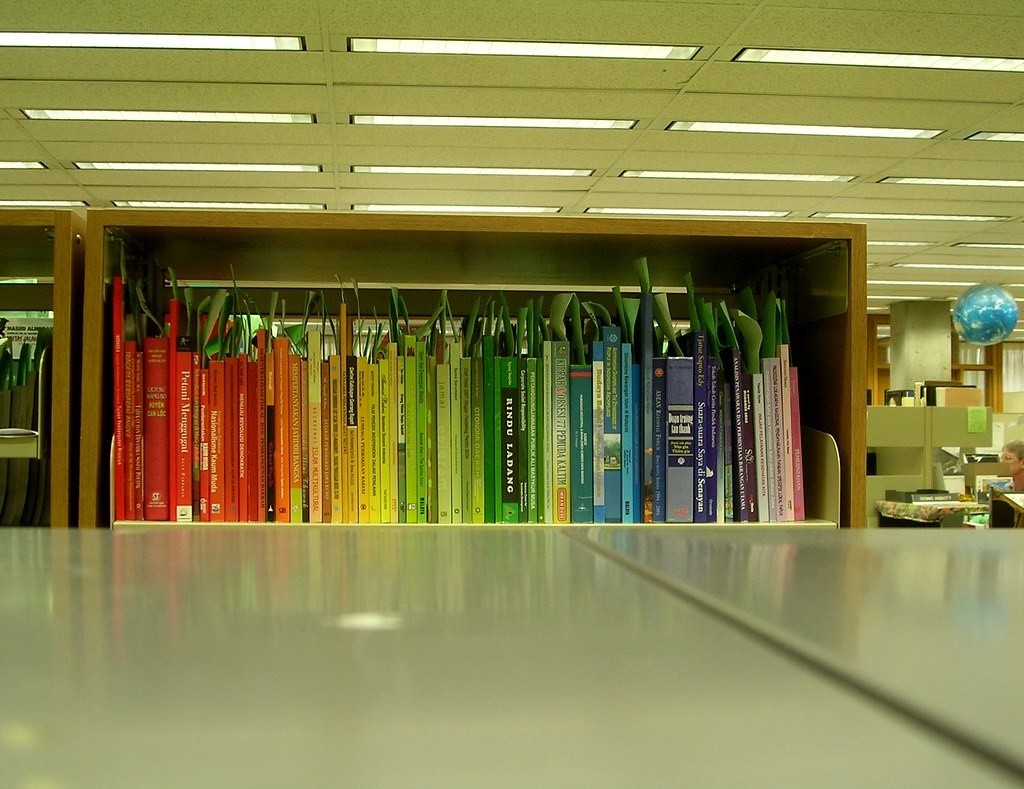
[0,204,869,530]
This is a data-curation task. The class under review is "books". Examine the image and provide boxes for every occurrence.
[3,337,50,525]
[111,276,808,523]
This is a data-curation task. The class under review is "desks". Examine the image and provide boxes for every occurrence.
[874,500,990,528]
[1,527,1024,789]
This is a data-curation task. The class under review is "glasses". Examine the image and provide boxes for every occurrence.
[999,455,1019,463]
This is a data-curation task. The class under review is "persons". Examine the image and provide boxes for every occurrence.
[1001,439,1024,493]
